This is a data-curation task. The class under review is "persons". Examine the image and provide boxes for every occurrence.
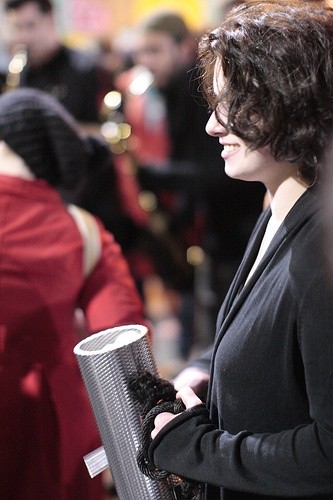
[150,0,333,500]
[0,0,333,500]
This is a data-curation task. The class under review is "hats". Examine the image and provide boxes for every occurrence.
[0,87,91,190]
